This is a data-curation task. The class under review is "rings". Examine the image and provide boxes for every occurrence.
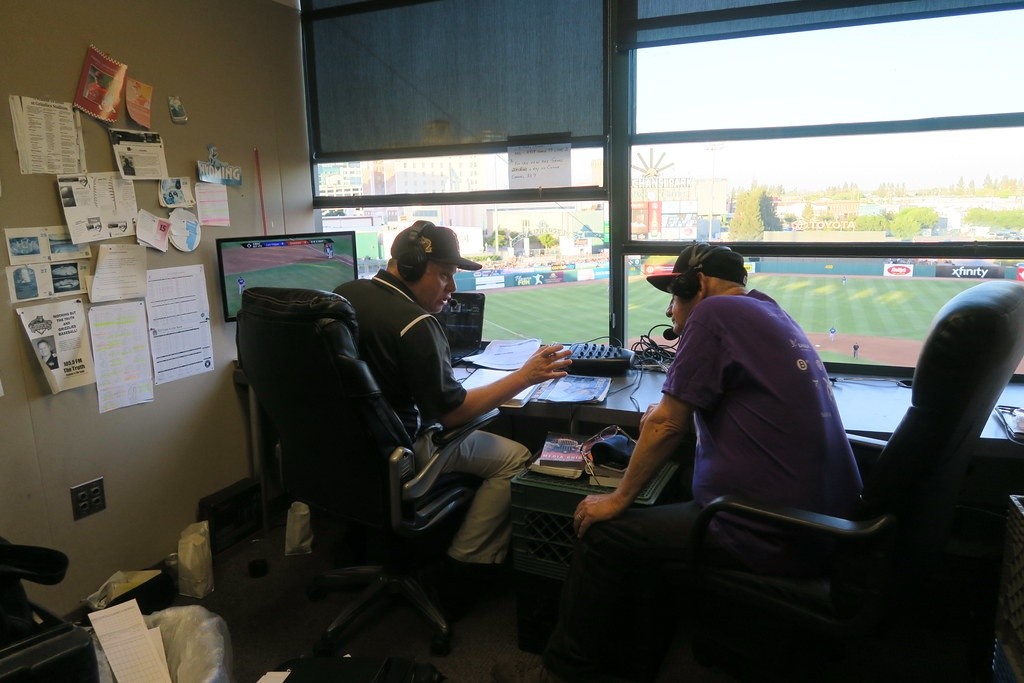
[578,515,583,521]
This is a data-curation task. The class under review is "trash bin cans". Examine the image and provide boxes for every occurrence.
[105,605,223,683]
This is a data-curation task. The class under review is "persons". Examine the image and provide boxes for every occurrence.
[238,276,245,295]
[332,227,572,563]
[326,243,334,259]
[491,243,864,683]
[37,340,59,370]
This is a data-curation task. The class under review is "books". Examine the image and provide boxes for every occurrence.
[461,368,611,408]
[531,432,628,487]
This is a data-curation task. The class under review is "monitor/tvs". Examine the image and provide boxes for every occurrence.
[215,231,358,323]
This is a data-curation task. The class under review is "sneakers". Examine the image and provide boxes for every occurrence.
[490,660,559,682]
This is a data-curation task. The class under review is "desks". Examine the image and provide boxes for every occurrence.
[230,338,1024,593]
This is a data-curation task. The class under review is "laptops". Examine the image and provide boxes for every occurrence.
[432,292,485,366]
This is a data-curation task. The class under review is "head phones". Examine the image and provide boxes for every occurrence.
[397,221,435,282]
[674,243,732,298]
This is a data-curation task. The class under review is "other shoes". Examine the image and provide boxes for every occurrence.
[414,559,497,603]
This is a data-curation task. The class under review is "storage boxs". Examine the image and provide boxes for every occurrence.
[991,635,1017,682]
[514,570,686,682]
[512,453,679,583]
[993,494,1024,683]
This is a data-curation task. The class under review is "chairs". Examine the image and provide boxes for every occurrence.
[236,287,502,658]
[675,281,1024,683]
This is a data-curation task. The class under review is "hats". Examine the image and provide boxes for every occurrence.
[646,243,748,292]
[390,226,483,271]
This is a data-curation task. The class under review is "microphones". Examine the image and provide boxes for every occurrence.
[663,328,679,340]
[449,297,458,307]
